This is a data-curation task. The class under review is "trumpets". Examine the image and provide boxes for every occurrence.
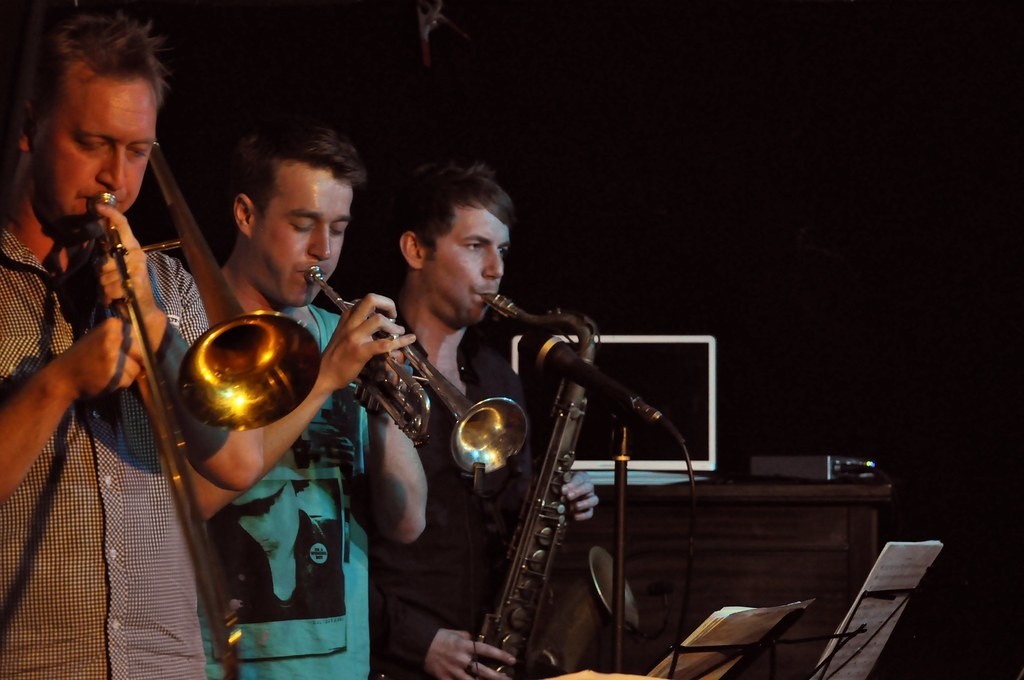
[306,261,529,478]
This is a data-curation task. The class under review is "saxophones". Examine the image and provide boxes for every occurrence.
[457,285,642,680]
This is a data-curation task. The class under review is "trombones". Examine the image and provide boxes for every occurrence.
[90,144,323,680]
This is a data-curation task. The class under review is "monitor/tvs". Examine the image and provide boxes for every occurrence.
[548,333,716,485]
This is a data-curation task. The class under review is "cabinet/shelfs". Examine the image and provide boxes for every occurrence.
[505,476,892,680]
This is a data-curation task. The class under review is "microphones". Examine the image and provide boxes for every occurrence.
[517,324,686,447]
[626,578,674,638]
[470,456,522,500]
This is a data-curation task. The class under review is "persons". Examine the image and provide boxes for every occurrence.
[0,11,211,680]
[369,162,599,680]
[188,123,427,680]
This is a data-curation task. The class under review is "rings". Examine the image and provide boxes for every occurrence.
[466,660,475,674]
